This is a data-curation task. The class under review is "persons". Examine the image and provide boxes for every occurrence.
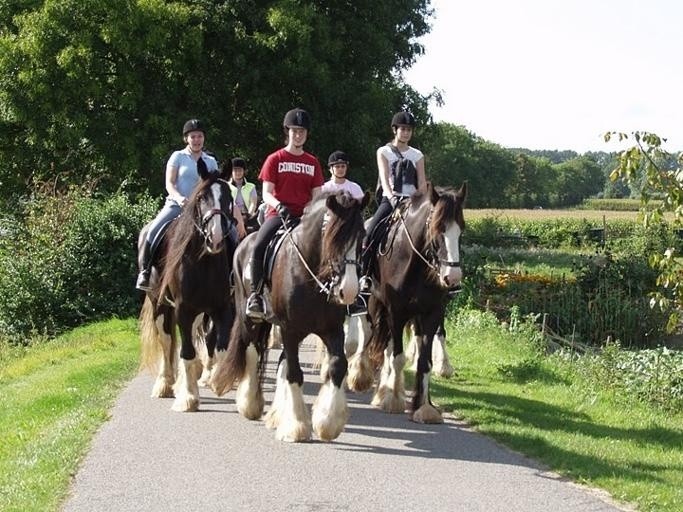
[322,152,364,201]
[358,113,424,291]
[246,109,325,322]
[228,158,256,231]
[136,120,219,289]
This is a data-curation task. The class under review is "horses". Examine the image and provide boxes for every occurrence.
[211,189,371,443]
[344,314,453,377]
[137,157,234,412]
[364,180,467,424]
[192,210,280,371]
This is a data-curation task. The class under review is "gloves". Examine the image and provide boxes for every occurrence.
[275,202,289,220]
[388,195,400,210]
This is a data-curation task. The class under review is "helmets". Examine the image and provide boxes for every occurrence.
[284,108,312,131]
[391,111,417,128]
[231,157,246,170]
[182,119,207,135]
[328,150,350,165]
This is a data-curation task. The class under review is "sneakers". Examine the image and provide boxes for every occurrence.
[137,270,149,287]
[359,275,372,295]
[247,291,264,324]
[449,285,462,295]
[345,303,368,317]
